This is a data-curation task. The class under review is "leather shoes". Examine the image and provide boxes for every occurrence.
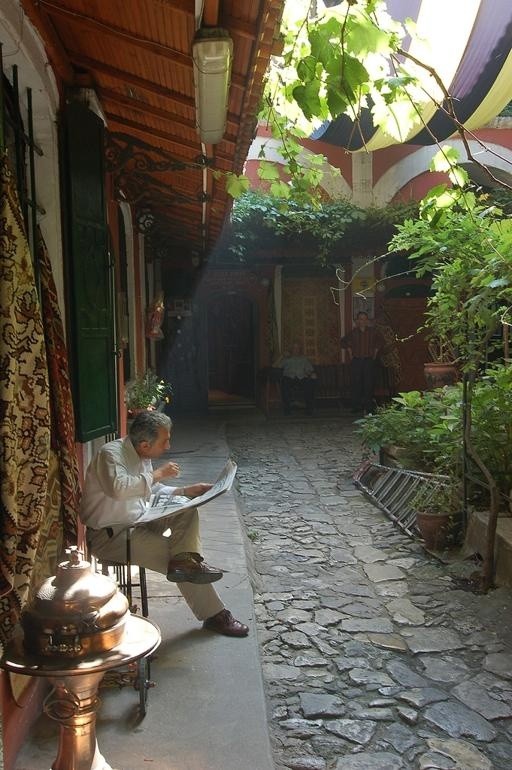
[165,556,224,585]
[202,608,250,636]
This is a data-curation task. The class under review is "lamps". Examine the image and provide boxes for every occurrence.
[191,27,234,145]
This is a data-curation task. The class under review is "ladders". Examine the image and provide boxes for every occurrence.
[353,461,452,540]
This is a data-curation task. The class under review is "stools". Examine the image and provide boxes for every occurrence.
[101,559,148,619]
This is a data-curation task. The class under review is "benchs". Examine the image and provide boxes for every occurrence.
[279,362,396,413]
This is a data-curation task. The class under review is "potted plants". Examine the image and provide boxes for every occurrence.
[422,344,460,389]
[414,481,465,548]
[125,379,152,420]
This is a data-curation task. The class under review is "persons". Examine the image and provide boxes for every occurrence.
[78,409,249,637]
[273,339,317,417]
[338,311,386,406]
